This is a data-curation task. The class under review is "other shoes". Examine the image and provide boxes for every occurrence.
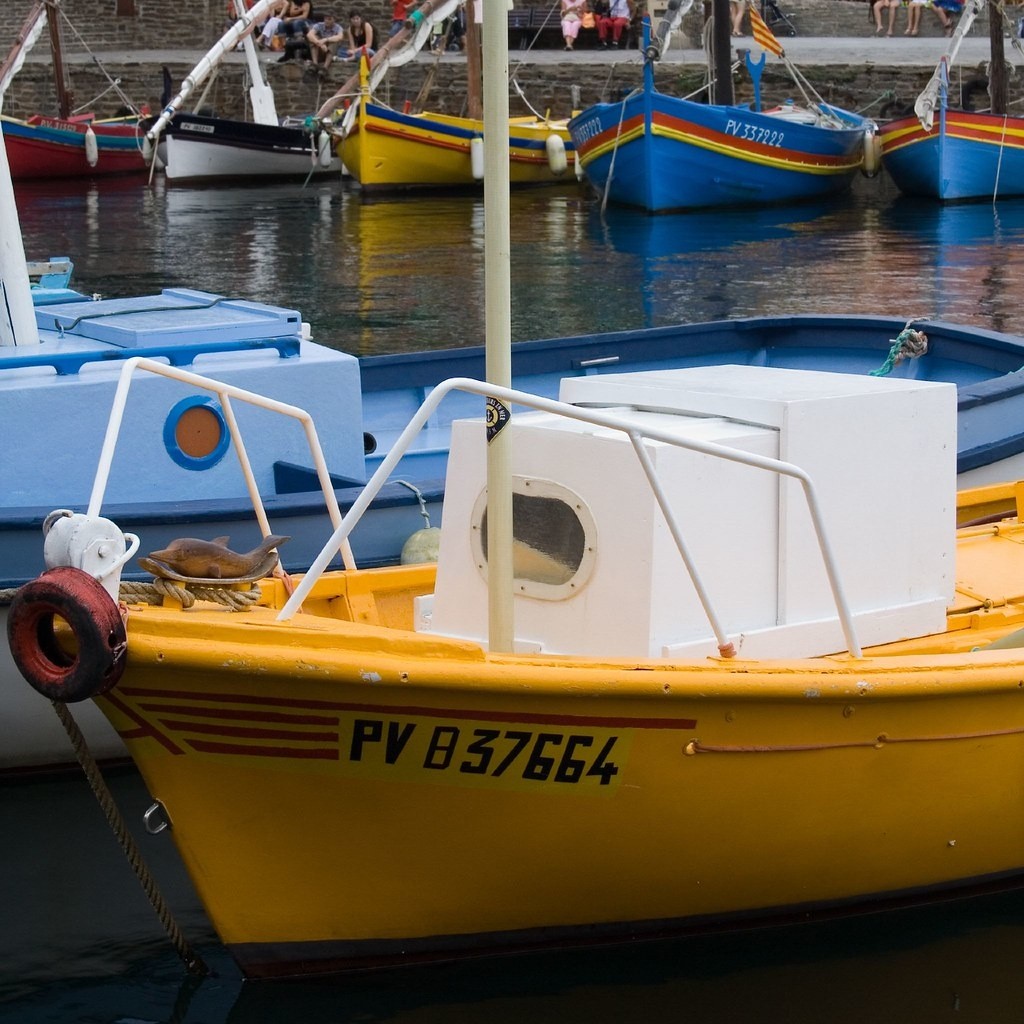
[904,28,912,35]
[612,41,618,46]
[452,41,464,50]
[256,41,265,50]
[944,19,954,37]
[319,67,327,77]
[237,48,245,52]
[267,45,275,52]
[911,29,918,35]
[309,63,319,73]
[564,44,573,51]
[731,31,744,37]
[332,55,340,61]
[602,41,607,48]
[886,30,894,35]
[873,27,883,36]
[429,50,439,55]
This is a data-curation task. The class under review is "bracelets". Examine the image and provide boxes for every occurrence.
[327,37,329,42]
[629,18,632,21]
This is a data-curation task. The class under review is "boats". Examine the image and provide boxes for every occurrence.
[1,85,1024,785]
[135,0,354,191]
[876,0,1024,205]
[1,0,1024,986]
[565,0,879,218]
[308,0,588,198]
[0,0,163,183]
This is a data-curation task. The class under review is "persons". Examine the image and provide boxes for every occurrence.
[598,0,636,49]
[932,0,965,36]
[453,4,467,56]
[332,10,379,62]
[873,0,900,34]
[729,0,746,38]
[307,8,343,76]
[560,0,588,51]
[388,0,418,44]
[223,0,313,51]
[904,0,931,35]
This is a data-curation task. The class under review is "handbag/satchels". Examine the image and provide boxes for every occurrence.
[581,11,595,28]
[607,10,611,18]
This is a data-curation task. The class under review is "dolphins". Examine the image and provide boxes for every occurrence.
[149,535,292,579]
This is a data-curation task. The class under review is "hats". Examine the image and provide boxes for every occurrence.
[323,8,336,16]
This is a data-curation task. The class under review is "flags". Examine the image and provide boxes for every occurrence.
[749,5,784,56]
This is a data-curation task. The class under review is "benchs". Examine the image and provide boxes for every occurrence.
[451,5,642,51]
